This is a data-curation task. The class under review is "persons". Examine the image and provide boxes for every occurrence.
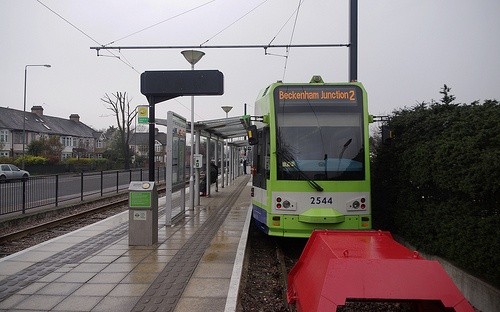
[199,164,217,196]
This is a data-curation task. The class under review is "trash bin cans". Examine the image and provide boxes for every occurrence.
[127,180,159,246]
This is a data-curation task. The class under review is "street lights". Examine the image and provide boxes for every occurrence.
[221,106,234,187]
[180,49,205,211]
[22,64,52,213]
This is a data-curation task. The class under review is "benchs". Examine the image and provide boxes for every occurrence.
[203,175,219,195]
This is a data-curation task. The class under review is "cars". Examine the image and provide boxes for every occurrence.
[0,163,31,185]
[202,156,243,171]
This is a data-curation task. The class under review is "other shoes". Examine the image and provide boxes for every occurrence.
[200,192,206,196]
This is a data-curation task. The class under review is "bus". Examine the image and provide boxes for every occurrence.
[240,74,393,239]
